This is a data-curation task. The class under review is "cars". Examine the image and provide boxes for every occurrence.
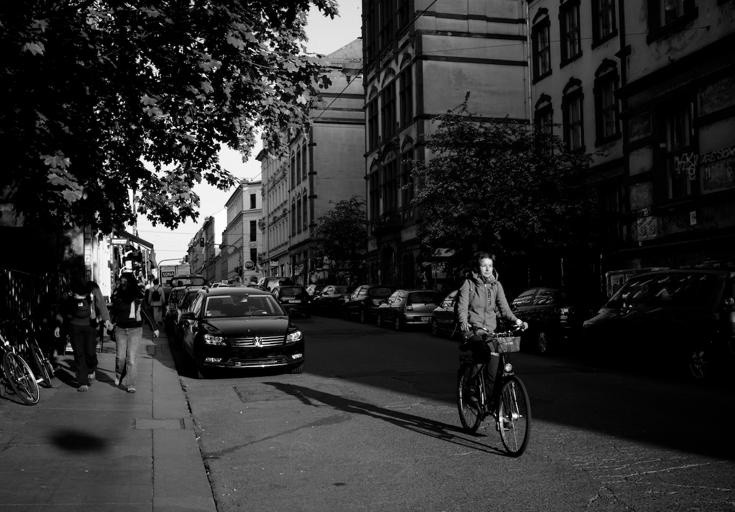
[163,274,461,378]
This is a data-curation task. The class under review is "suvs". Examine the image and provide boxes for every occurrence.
[502,285,576,359]
[579,268,735,379]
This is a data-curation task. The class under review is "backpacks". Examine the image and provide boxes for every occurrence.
[150,287,160,303]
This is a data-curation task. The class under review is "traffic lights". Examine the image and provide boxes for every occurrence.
[185,255,188,262]
[200,237,204,247]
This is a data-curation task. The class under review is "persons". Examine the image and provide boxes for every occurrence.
[109,273,159,394]
[452,250,528,432]
[147,279,165,330]
[54,279,114,393]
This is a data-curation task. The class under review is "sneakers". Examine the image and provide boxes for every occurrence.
[77,383,87,392]
[113,378,121,386]
[87,372,94,380]
[126,386,136,393]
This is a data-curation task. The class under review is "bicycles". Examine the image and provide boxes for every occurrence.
[455,320,534,456]
[0,316,56,407]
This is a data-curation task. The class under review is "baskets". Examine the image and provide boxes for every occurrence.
[493,337,521,353]
